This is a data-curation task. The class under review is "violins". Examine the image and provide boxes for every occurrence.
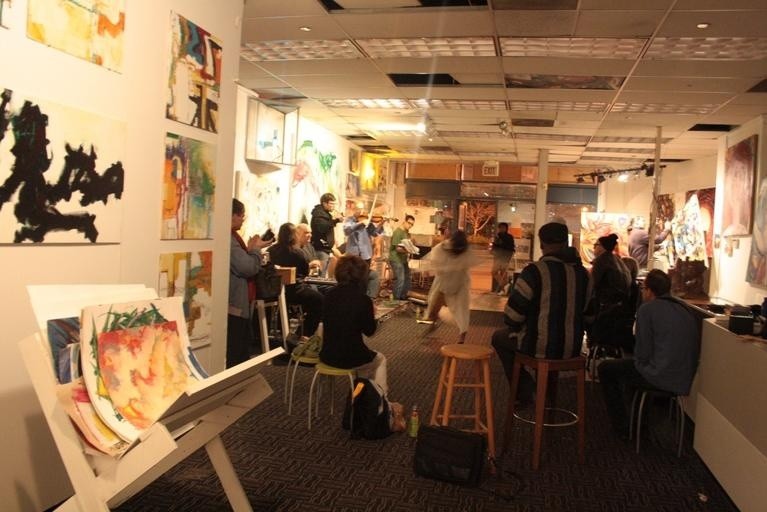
[356,211,399,222]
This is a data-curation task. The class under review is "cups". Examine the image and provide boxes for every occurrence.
[309,264,320,278]
[260,252,270,265]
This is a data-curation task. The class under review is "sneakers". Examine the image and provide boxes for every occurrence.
[503,283,510,295]
[496,288,504,296]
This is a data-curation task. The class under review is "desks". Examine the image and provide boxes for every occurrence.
[304,277,338,287]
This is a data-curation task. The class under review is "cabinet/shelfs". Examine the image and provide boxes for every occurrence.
[245,97,300,167]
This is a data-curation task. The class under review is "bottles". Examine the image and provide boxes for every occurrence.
[415,306,421,319]
[408,404,421,439]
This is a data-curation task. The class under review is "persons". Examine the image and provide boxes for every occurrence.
[571,233,633,358]
[226,199,274,370]
[597,270,699,440]
[420,219,485,345]
[615,256,643,353]
[628,217,671,279]
[266,192,390,404]
[491,222,593,409]
[388,215,416,300]
[490,222,515,296]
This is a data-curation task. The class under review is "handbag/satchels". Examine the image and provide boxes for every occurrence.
[294,336,320,358]
[256,261,282,299]
[413,423,491,488]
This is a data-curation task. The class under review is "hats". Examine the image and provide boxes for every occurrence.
[539,222,568,244]
[598,234,618,252]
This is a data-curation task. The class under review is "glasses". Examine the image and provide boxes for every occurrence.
[639,284,649,290]
[407,221,413,226]
[594,242,602,248]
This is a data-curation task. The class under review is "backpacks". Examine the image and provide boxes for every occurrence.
[343,378,393,440]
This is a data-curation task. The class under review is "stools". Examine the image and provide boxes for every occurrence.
[428,343,496,459]
[275,303,304,338]
[628,387,685,458]
[308,362,358,432]
[586,341,624,393]
[506,349,586,470]
[284,353,335,416]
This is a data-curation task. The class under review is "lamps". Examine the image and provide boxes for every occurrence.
[598,175,606,183]
[499,121,508,136]
[577,176,584,182]
[641,164,654,176]
